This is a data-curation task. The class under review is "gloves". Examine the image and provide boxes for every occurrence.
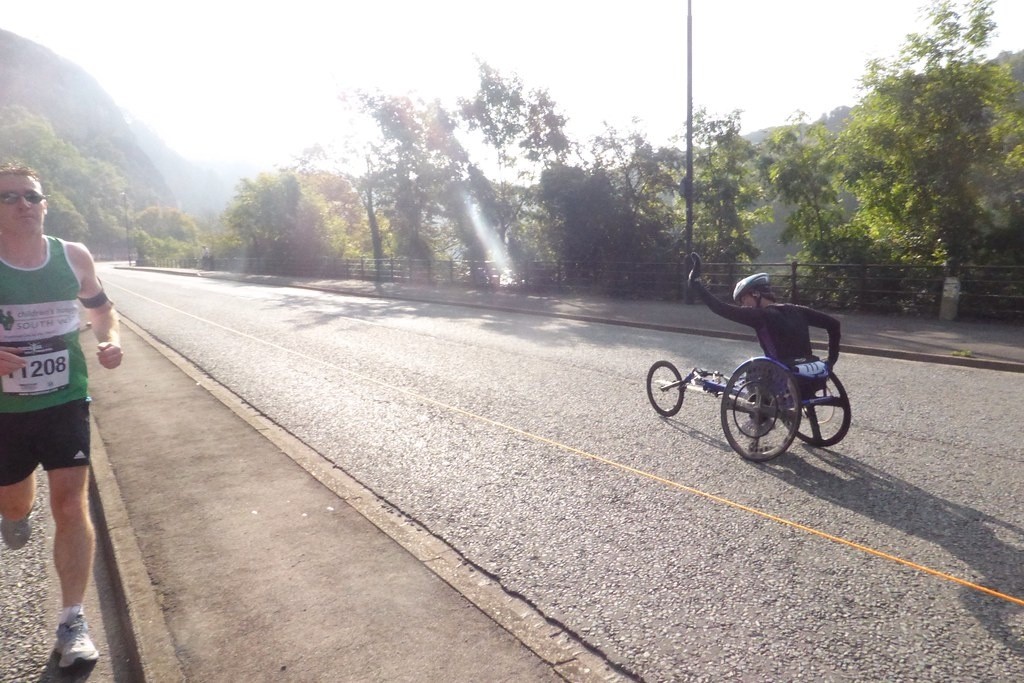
[685,252,703,288]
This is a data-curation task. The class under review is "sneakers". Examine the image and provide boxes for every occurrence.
[0,515,32,549]
[53,604,99,667]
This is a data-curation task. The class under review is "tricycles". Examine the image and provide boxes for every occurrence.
[646,353,852,462]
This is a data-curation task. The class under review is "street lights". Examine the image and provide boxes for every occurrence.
[119,191,132,266]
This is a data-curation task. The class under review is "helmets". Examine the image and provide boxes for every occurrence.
[733,273,770,305]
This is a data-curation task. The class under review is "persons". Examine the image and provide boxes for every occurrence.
[199,244,210,270]
[688,251,842,392]
[0,160,125,674]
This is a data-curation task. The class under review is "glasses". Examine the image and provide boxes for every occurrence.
[0,189,51,204]
